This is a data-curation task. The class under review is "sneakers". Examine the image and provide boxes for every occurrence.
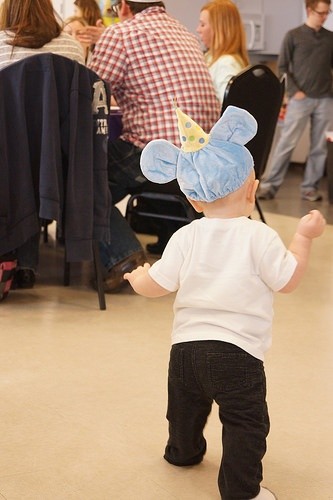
[93,252,147,293]
[257,190,273,199]
[302,190,322,201]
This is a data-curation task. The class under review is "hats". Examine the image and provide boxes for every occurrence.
[140,96,258,202]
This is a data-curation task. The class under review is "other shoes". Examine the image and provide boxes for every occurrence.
[146,244,161,254]
[249,486,277,500]
[3,269,35,289]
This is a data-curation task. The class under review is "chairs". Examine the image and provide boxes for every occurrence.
[122,65,286,236]
[0,53,111,311]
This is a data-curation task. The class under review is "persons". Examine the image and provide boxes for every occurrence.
[87,0,221,292]
[196,0,254,104]
[0,0,122,291]
[123,134,326,500]
[255,0,333,202]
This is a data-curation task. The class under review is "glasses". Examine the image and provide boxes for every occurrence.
[111,0,131,15]
[312,9,329,15]
